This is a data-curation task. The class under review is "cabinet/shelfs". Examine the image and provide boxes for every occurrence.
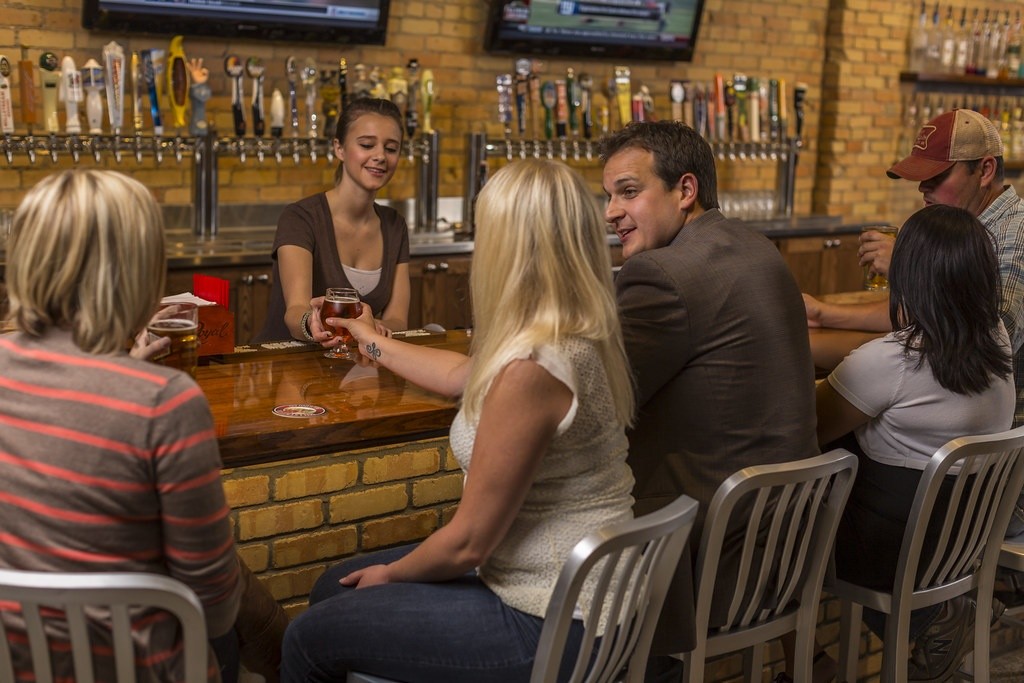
[157,254,472,350]
[774,231,873,298]
[902,71,1024,175]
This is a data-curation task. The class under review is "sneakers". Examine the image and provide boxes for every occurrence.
[909,595,1006,683]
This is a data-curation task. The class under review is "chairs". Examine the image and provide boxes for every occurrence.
[665,448,860,682]
[0,569,212,682]
[826,427,1024,682]
[345,497,700,683]
[958,539,1024,644]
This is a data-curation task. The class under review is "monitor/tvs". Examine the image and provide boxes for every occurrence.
[81,0,390,46]
[483,0,705,62]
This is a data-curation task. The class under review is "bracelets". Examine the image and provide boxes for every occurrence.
[301,309,313,341]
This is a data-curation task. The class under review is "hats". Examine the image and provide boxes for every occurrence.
[886,109,1004,181]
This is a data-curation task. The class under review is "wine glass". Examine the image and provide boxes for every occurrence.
[320,287,364,359]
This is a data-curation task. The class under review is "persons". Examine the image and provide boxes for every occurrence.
[805,206,1015,593]
[0,168,287,683]
[857,108,1024,683]
[278,161,640,682]
[600,119,818,683]
[261,96,410,344]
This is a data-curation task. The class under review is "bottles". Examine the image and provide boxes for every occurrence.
[953,8,969,77]
[941,6,955,77]
[978,8,991,76]
[925,3,942,79]
[967,8,981,75]
[896,88,932,159]
[998,9,1011,79]
[962,93,979,112]
[909,0,927,72]
[936,92,961,116]
[998,98,1024,169]
[1006,11,1023,79]
[986,11,1001,79]
[982,95,1000,132]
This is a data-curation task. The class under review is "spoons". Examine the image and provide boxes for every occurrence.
[301,58,318,161]
[247,56,266,162]
[287,56,299,162]
[226,54,246,163]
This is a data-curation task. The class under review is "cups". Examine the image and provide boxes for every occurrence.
[717,192,776,220]
[145,302,198,381]
[862,227,898,290]
[0,207,14,250]
